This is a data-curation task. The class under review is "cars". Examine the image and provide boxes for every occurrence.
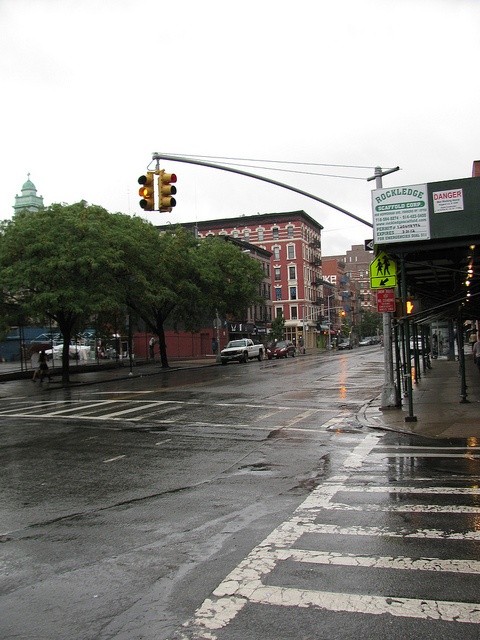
[358,338,374,346]
[267,340,296,359]
[337,338,353,351]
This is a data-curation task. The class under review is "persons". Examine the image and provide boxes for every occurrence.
[39,350,53,382]
[31,351,41,382]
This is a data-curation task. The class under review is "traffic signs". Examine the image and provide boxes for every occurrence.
[369,250,397,289]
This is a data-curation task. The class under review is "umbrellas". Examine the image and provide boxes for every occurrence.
[30,344,53,351]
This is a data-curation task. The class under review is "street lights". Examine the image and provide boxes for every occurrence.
[327,290,349,351]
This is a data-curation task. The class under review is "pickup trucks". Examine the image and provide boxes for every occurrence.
[220,338,266,365]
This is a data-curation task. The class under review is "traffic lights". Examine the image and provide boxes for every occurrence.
[138,171,155,211]
[406,300,419,314]
[341,310,346,317]
[158,169,177,212]
